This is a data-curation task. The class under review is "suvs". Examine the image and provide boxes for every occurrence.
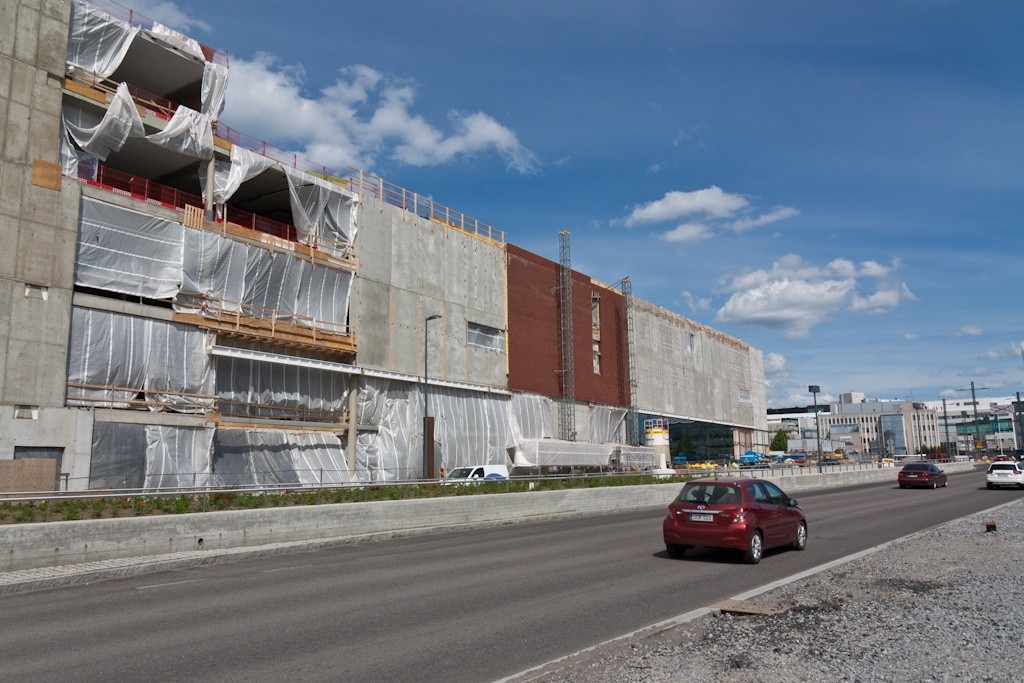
[985,460,1024,490]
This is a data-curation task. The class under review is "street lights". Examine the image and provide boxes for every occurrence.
[955,381,990,460]
[424,314,442,479]
[912,402,923,461]
[808,385,821,466]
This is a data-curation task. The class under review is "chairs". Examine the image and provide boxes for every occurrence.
[707,486,729,504]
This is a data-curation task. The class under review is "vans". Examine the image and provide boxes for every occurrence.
[439,464,510,488]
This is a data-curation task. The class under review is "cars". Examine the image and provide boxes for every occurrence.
[994,455,1013,461]
[816,458,840,466]
[898,463,948,489]
[663,476,808,563]
